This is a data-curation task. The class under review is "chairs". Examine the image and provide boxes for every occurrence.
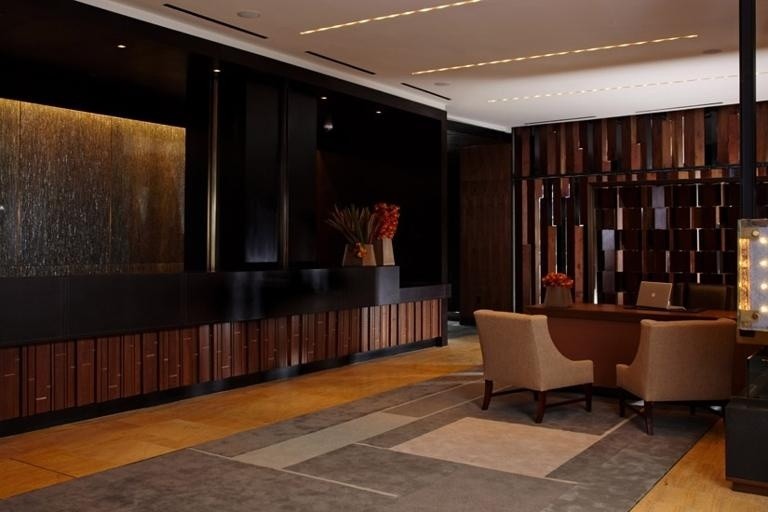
[473,309,594,423]
[615,317,737,436]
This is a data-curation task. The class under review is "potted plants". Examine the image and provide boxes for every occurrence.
[324,203,378,267]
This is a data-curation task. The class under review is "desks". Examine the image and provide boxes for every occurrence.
[522,302,738,389]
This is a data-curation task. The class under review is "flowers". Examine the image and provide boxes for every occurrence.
[371,200,400,240]
[541,272,573,290]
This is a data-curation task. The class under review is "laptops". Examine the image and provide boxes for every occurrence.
[636,281,683,310]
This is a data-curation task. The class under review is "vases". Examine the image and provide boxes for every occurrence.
[543,287,573,306]
[372,236,396,266]
